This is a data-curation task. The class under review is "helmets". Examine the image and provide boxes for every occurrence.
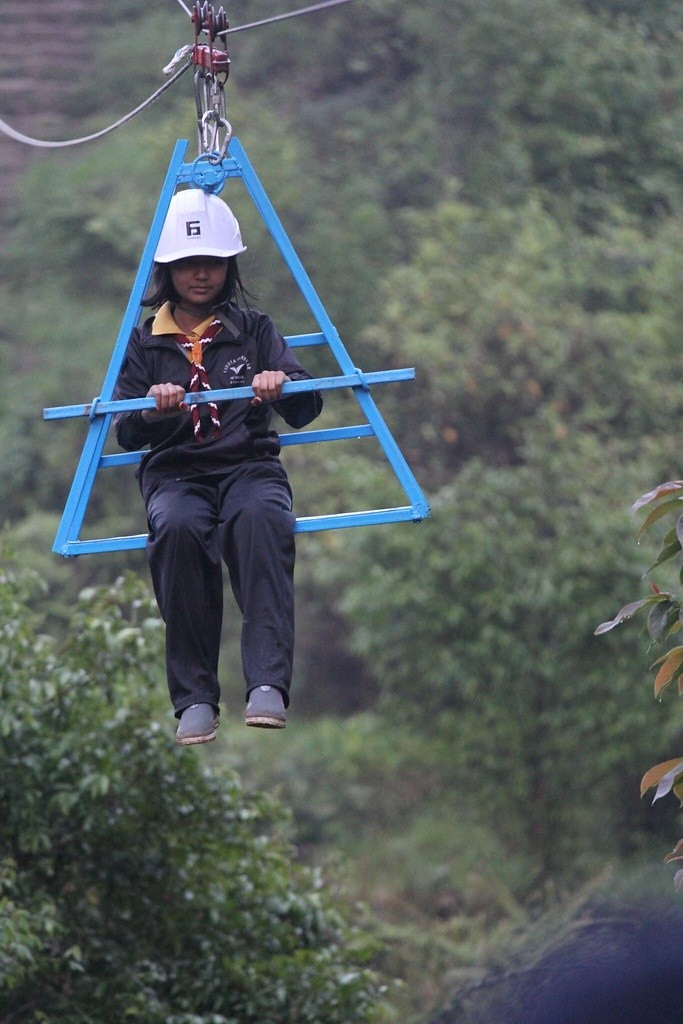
[153,188,247,264]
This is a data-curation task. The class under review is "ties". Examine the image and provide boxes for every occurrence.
[172,317,225,441]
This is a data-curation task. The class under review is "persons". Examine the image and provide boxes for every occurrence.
[116,188,331,741]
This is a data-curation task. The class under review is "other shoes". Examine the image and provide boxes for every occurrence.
[175,703,220,745]
[245,685,286,729]
